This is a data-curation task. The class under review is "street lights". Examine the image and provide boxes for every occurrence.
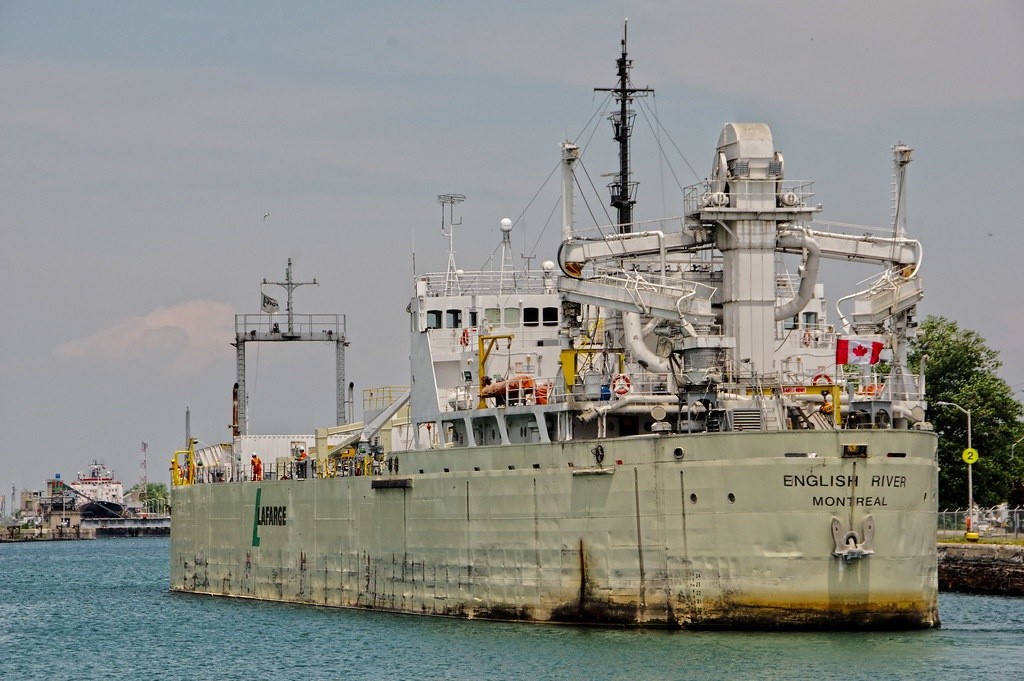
[937,401,974,532]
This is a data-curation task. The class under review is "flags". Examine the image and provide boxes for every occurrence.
[261,292,280,313]
[835,339,884,365]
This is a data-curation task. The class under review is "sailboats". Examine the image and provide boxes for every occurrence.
[173,18,949,632]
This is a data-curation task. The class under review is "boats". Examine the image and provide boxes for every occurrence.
[67,459,172,537]
[0,472,96,542]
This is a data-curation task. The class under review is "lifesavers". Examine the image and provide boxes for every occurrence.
[462,328,469,346]
[612,375,631,395]
[813,373,832,386]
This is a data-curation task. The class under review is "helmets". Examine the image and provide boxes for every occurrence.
[299,446,305,450]
[252,452,256,456]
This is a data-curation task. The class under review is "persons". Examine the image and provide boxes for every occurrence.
[298,446,309,461]
[170,458,195,484]
[251,452,262,482]
